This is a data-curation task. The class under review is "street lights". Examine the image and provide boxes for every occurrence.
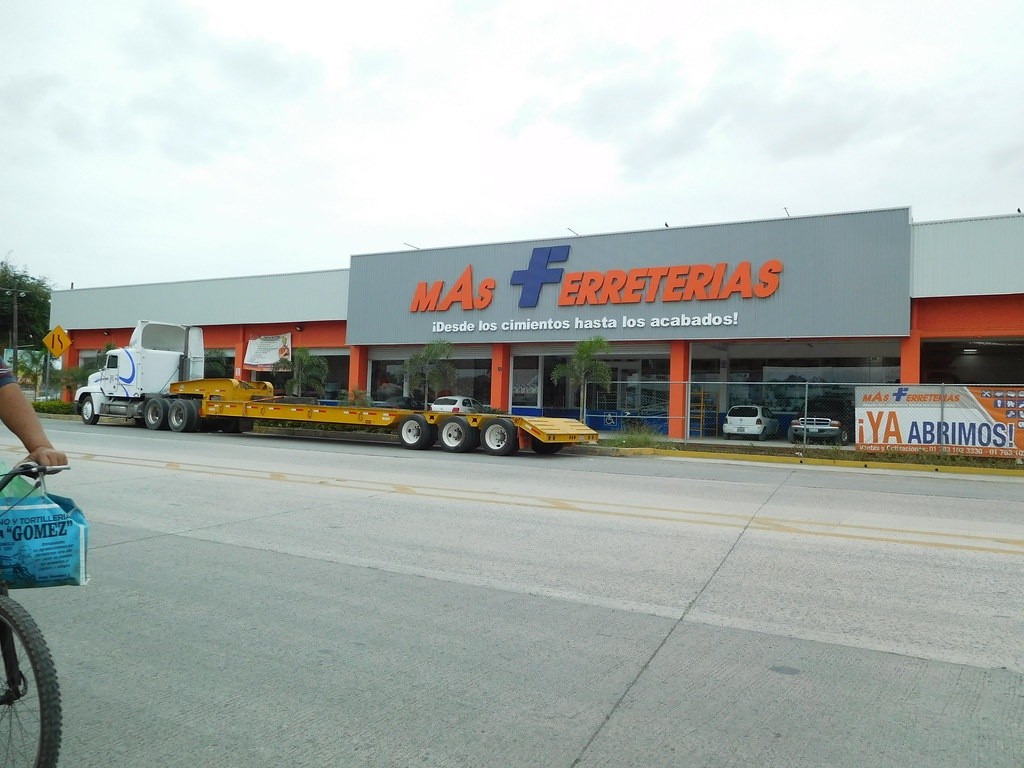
[6,288,26,380]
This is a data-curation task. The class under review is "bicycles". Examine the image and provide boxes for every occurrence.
[0,463,71,768]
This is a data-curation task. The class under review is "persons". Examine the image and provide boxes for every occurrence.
[0,357,68,474]
[278,337,290,359]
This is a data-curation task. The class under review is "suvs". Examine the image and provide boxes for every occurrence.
[787,398,855,445]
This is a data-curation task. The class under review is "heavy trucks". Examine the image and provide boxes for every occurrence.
[75,319,598,457]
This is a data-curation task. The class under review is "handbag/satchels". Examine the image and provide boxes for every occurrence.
[0,461,92,588]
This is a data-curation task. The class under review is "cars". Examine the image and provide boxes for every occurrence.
[723,404,780,441]
[429,396,486,414]
[380,396,423,410]
